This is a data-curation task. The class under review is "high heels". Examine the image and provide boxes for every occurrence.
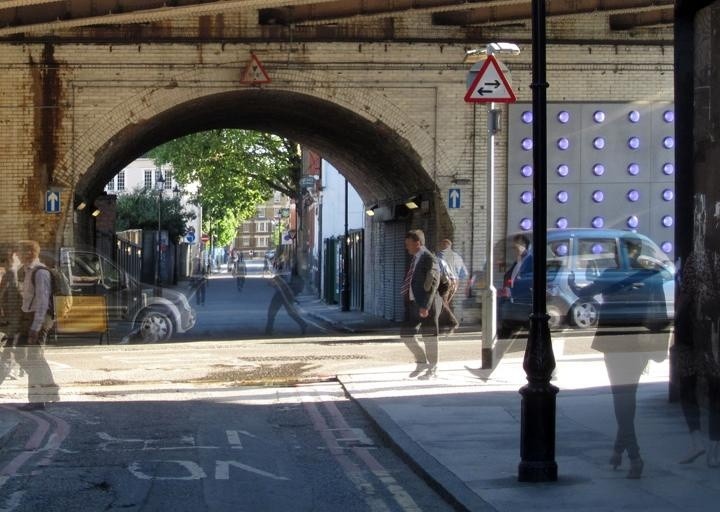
[610,456,644,478]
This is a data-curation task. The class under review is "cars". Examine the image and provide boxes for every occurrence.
[465,227,674,339]
[51,245,198,345]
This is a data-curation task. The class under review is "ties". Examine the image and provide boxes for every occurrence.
[401,257,415,295]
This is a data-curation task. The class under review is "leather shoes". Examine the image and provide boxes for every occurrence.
[18,399,58,410]
[678,448,717,468]
[464,364,489,381]
[410,366,437,379]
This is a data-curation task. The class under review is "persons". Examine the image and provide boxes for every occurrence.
[566,230,672,483]
[398,227,444,382]
[192,257,208,303]
[13,238,62,411]
[261,239,308,334]
[0,241,30,385]
[463,234,534,382]
[433,236,472,339]
[220,244,272,292]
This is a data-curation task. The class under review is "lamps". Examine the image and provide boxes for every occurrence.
[73,199,101,219]
[366,195,421,216]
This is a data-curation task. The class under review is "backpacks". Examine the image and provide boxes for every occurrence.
[29,268,73,318]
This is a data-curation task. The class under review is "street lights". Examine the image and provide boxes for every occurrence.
[462,40,524,374]
[173,183,180,287]
[276,208,283,271]
[154,173,166,286]
